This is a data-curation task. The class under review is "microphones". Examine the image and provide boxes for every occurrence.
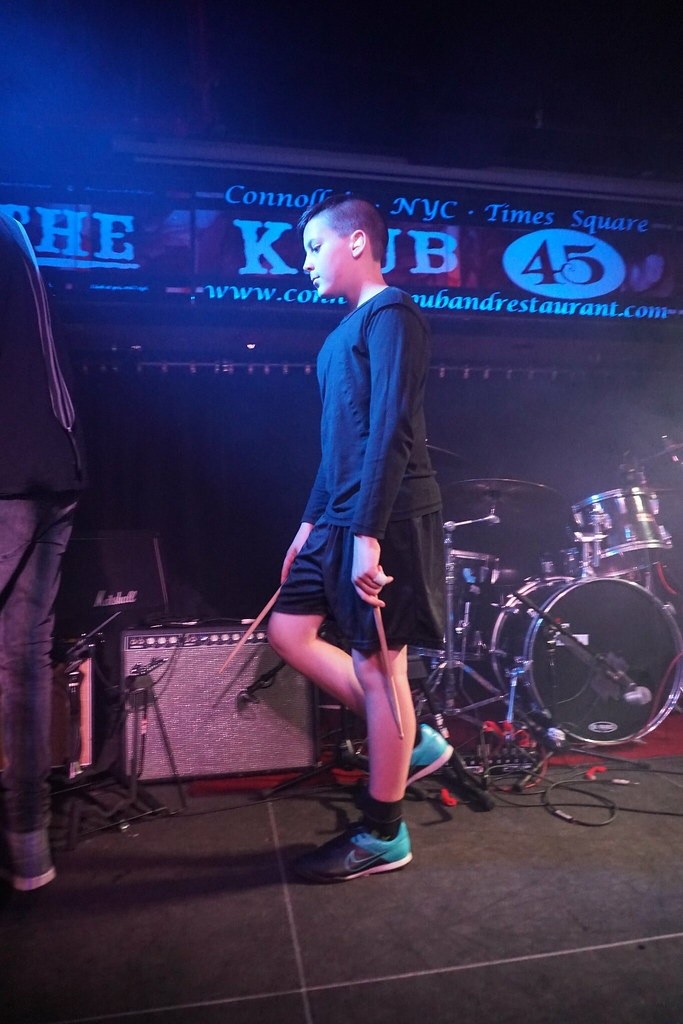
[239,690,260,705]
[625,686,653,705]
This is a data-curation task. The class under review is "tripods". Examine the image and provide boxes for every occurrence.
[93,657,186,811]
[258,501,652,801]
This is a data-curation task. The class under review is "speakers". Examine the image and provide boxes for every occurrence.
[121,618,321,785]
[51,528,169,637]
[0,637,95,774]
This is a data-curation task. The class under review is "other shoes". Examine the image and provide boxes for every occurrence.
[0,828,58,892]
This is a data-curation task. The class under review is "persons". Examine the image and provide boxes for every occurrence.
[0,214,85,887]
[267,201,454,883]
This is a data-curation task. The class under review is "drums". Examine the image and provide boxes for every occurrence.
[568,485,675,579]
[444,547,502,603]
[537,545,583,581]
[489,574,683,747]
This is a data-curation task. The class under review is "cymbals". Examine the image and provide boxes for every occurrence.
[427,442,464,461]
[640,442,683,463]
[442,476,570,518]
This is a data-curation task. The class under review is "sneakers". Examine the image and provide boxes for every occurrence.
[353,723,455,810]
[298,820,413,883]
